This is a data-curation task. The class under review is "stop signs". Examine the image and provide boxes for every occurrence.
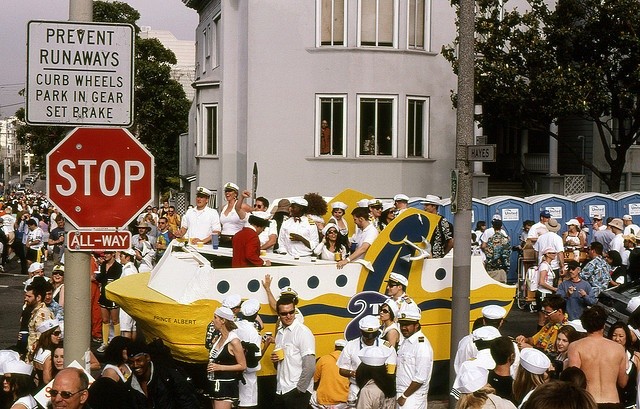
[47,127,154,231]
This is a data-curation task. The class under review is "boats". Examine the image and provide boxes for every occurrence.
[105,189,518,379]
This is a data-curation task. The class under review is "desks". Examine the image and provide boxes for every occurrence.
[184,243,344,268]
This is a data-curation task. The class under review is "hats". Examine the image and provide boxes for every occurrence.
[359,315,381,339]
[368,199,383,207]
[0,350,34,376]
[382,203,396,212]
[28,262,44,272]
[52,264,64,273]
[482,305,506,320]
[332,201,348,210]
[594,214,602,219]
[566,219,580,228]
[280,286,298,296]
[128,348,147,358]
[540,210,550,218]
[222,294,241,309]
[121,248,135,256]
[456,361,490,393]
[520,347,551,375]
[621,215,632,220]
[196,186,211,197]
[249,212,272,227]
[135,223,150,228]
[322,223,339,235]
[239,299,261,317]
[397,304,421,325]
[569,260,580,266]
[214,307,234,322]
[420,195,443,206]
[491,214,502,222]
[335,339,347,348]
[358,346,392,367]
[622,234,636,245]
[275,199,291,214]
[224,182,239,192]
[384,299,399,319]
[357,199,370,207]
[36,319,59,334]
[544,218,561,232]
[290,197,308,207]
[394,194,409,205]
[635,230,640,240]
[384,272,408,287]
[543,247,557,254]
[473,326,502,341]
[608,218,624,231]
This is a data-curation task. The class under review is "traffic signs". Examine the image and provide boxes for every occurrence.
[465,137,503,166]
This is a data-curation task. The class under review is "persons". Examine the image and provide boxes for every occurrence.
[469,211,511,285]
[99,337,136,383]
[319,119,331,155]
[385,272,423,332]
[520,207,640,323]
[312,221,349,264]
[275,195,319,260]
[549,321,576,366]
[230,205,271,267]
[93,198,182,345]
[336,315,398,407]
[262,273,316,409]
[14,262,64,365]
[380,300,402,349]
[204,293,273,409]
[337,207,379,269]
[1,358,40,409]
[396,309,434,409]
[219,179,247,249]
[1,188,67,264]
[607,316,640,409]
[353,346,398,409]
[328,197,350,254]
[454,303,518,409]
[124,347,195,408]
[523,378,598,409]
[364,195,412,234]
[174,185,222,247]
[46,366,92,409]
[414,189,456,261]
[564,303,630,409]
[235,186,269,222]
[309,338,351,409]
[515,297,572,353]
[87,348,101,373]
[509,345,549,406]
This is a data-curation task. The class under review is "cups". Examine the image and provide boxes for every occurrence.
[335,251,340,261]
[310,253,317,261]
[289,230,295,240]
[387,362,396,375]
[211,232,220,250]
[197,241,203,248]
[260,250,266,257]
[263,331,273,342]
[273,348,284,361]
[295,252,299,260]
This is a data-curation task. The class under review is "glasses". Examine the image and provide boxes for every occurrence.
[380,307,390,314]
[594,219,602,221]
[279,307,295,316]
[158,222,164,224]
[254,204,262,208]
[53,272,64,276]
[51,330,61,336]
[2,375,12,382]
[373,206,383,210]
[139,239,143,241]
[50,388,84,400]
[541,310,558,316]
[389,209,397,214]
[327,230,338,234]
[147,209,151,210]
[169,210,174,212]
[387,284,393,288]
[566,267,580,271]
